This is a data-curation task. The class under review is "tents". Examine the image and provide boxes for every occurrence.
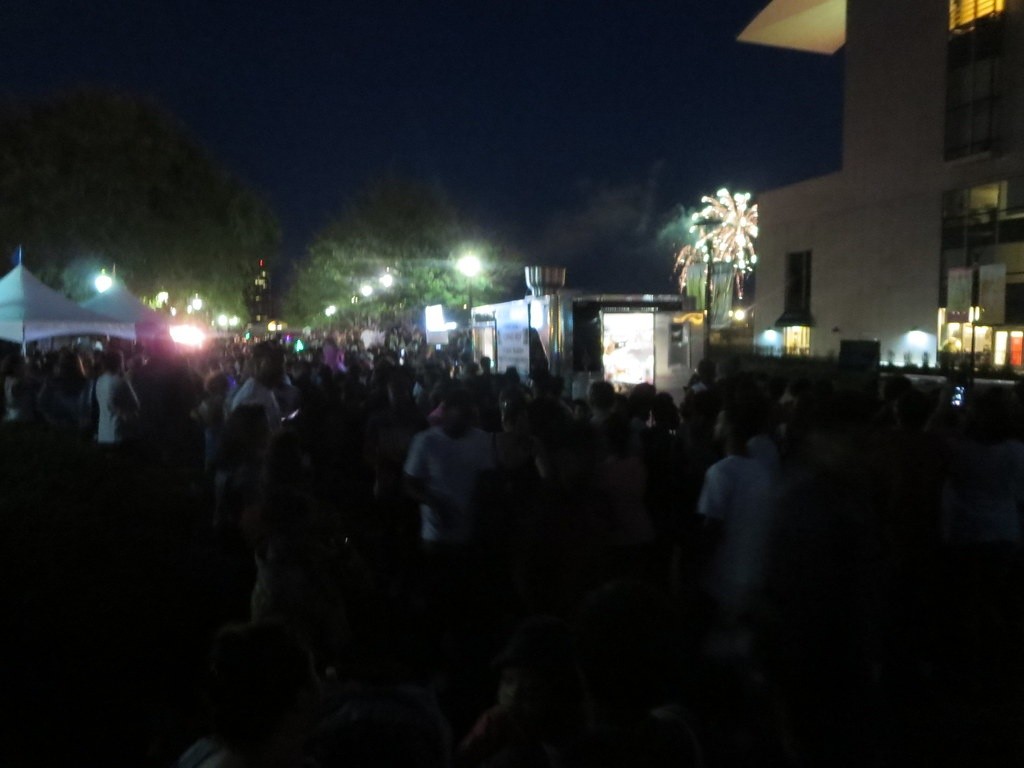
[0,246,174,348]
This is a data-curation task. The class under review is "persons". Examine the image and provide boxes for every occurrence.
[1,321,1024,768]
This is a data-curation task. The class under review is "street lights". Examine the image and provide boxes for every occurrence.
[325,305,335,336]
[218,315,228,351]
[695,204,723,361]
[268,322,277,343]
[157,291,169,335]
[382,274,394,331]
[95,275,111,347]
[192,296,203,350]
[451,247,486,353]
[360,286,373,330]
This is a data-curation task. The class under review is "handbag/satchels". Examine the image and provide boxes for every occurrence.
[109,386,139,418]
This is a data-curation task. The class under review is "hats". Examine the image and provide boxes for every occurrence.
[494,614,579,672]
[252,340,284,375]
